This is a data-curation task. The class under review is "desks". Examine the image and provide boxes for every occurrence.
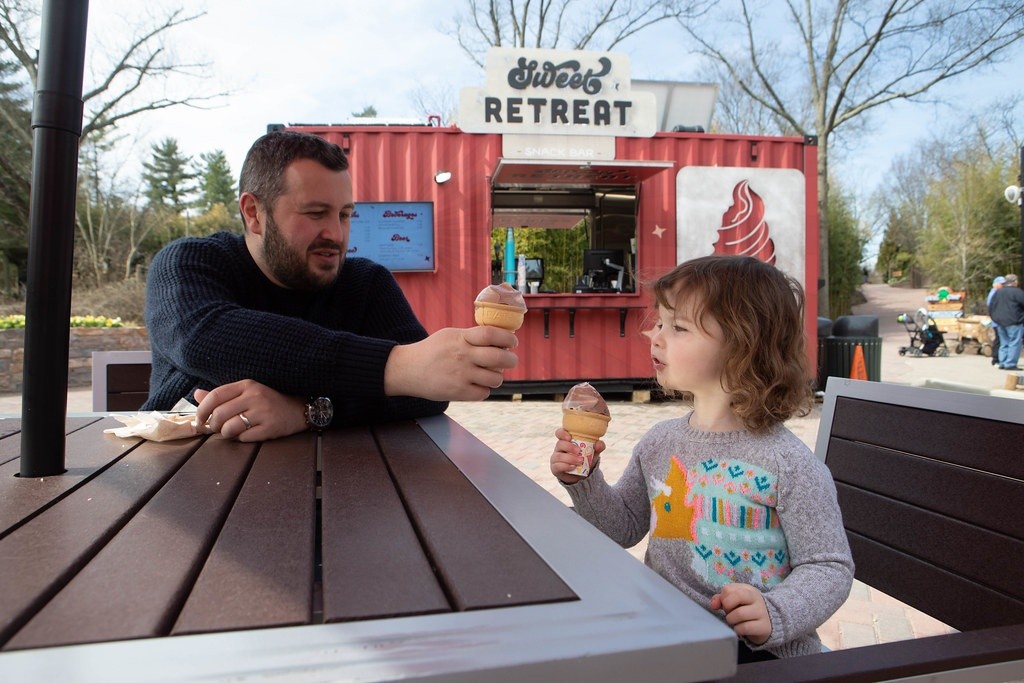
[0,411,737,682]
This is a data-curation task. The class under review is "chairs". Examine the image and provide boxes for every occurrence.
[736,375,1024,682]
[90,350,152,412]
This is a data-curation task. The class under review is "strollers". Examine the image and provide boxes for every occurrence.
[897,307,948,358]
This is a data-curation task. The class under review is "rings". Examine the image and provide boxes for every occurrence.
[238,412,251,430]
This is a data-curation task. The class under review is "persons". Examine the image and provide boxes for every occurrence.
[987,277,1005,368]
[549,254,856,666]
[139,131,519,441]
[988,272,1023,370]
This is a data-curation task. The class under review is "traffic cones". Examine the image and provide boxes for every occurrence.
[850,344,868,381]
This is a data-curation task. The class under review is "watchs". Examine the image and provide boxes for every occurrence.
[304,395,334,433]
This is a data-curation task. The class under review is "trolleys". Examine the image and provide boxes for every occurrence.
[956,316,997,357]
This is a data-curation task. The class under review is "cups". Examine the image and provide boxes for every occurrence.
[503,226,540,295]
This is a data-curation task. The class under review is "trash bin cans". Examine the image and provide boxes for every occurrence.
[825,314,882,382]
[815,316,834,393]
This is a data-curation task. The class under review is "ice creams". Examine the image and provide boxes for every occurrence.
[561,381,611,477]
[709,178,776,266]
[473,281,528,333]
